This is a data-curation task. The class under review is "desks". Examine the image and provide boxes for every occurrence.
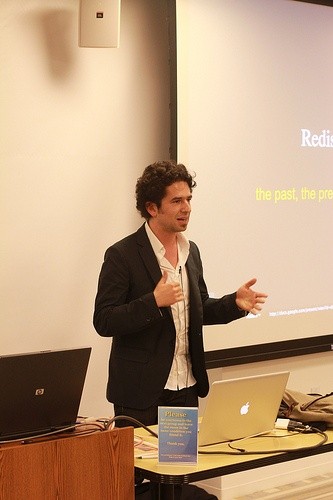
[133,417,333,500]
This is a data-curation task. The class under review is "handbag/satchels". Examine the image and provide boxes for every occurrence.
[277,388,333,428]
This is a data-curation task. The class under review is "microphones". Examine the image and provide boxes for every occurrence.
[179,266,181,274]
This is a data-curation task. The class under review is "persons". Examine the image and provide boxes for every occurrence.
[92,159,269,500]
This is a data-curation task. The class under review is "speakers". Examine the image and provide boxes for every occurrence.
[79,0,121,49]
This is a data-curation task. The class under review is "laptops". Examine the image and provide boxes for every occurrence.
[0,346,92,441]
[198,371,290,446]
